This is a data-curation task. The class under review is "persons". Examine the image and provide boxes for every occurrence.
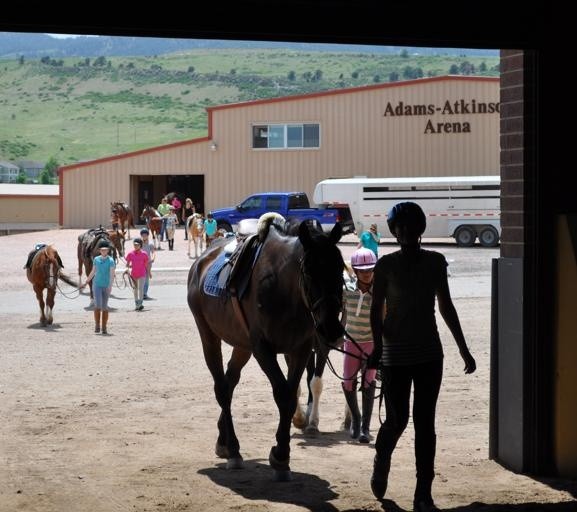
[182,198,195,240]
[356,223,381,258]
[172,194,181,227]
[152,206,179,251]
[202,214,219,248]
[125,239,152,311]
[140,227,155,299]
[80,240,115,335]
[156,198,171,242]
[339,247,377,443]
[370,201,477,512]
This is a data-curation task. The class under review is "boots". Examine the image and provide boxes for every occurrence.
[342,382,361,438]
[359,381,376,443]
[169,240,171,251]
[370,449,391,499]
[135,300,140,311]
[414,477,442,512]
[139,300,144,310]
[94,308,101,333]
[171,238,174,251]
[102,310,108,334]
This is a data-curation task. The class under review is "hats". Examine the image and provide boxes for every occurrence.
[98,241,110,249]
[352,248,378,271]
[169,206,175,210]
[133,239,143,244]
[388,203,426,237]
[207,212,213,218]
[140,228,149,235]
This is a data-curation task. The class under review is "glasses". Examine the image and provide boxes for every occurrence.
[369,228,375,231]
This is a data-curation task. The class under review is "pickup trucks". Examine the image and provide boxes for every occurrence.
[207,190,355,249]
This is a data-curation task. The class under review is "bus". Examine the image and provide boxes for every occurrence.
[312,175,501,246]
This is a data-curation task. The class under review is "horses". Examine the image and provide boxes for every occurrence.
[77,226,117,301]
[187,214,205,260]
[23,244,62,329]
[282,348,330,437]
[107,226,127,256]
[140,205,171,253]
[111,200,137,234]
[187,218,347,482]
